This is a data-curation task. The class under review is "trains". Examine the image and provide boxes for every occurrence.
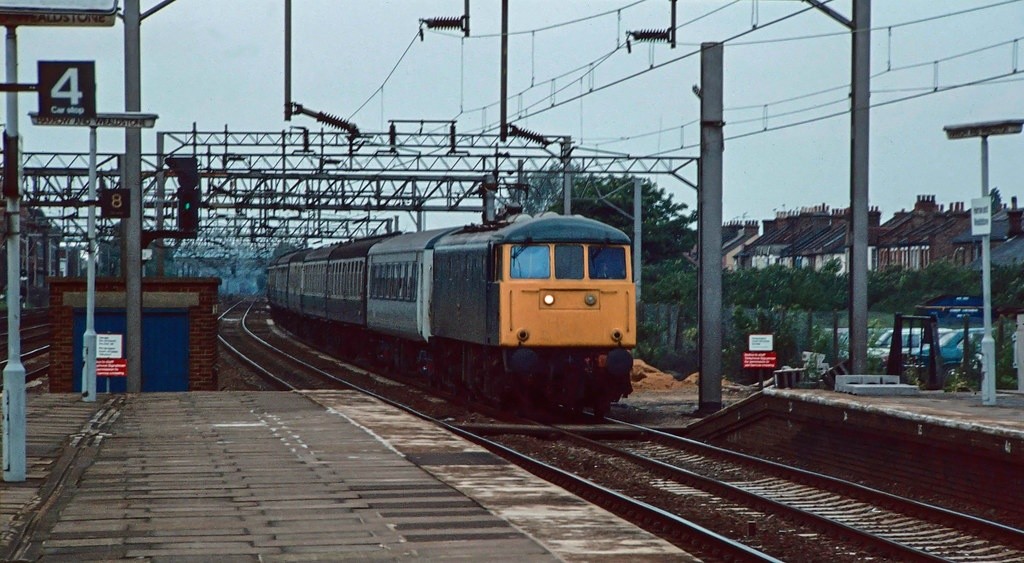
[263,210,639,419]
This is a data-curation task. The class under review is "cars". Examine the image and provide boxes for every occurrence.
[823,325,998,383]
